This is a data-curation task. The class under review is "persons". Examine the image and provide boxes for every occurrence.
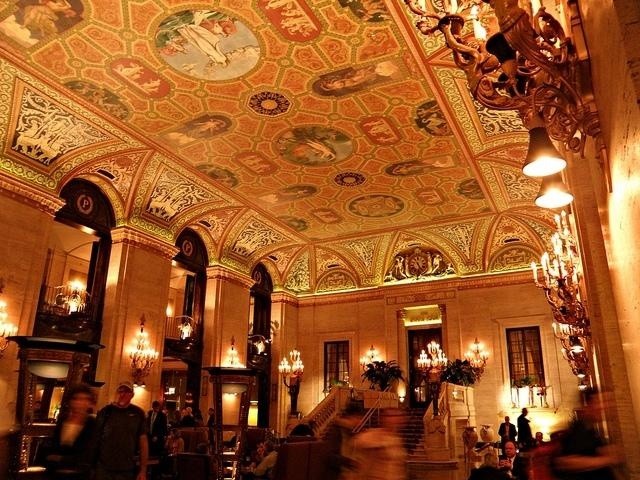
[54,381,216,480]
[476,407,539,480]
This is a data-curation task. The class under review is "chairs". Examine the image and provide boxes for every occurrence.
[0,425,340,480]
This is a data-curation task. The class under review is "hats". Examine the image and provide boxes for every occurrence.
[117,381,133,391]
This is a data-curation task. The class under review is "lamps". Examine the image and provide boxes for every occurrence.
[403,1,603,210]
[0,276,19,358]
[68,282,86,313]
[463,335,491,377]
[528,210,595,394]
[417,339,449,374]
[359,344,384,369]
[229,335,236,365]
[248,399,258,427]
[126,311,160,388]
[278,347,305,417]
[178,321,194,340]
